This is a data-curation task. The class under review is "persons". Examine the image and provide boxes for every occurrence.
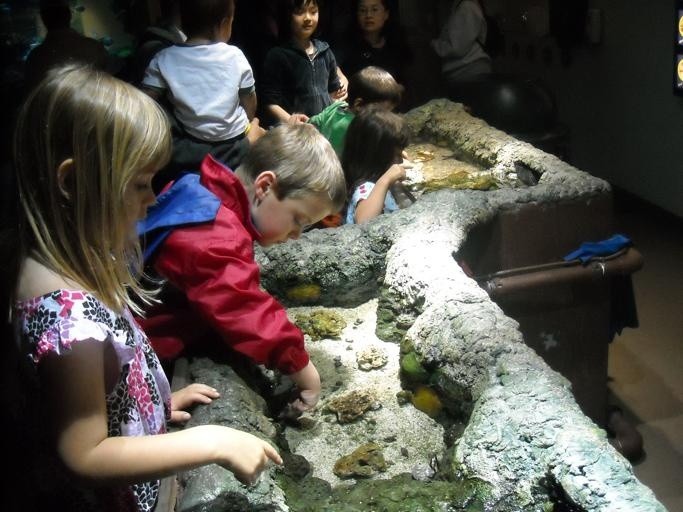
[125,122,347,417]
[3,62,284,511]
[2,1,494,226]
